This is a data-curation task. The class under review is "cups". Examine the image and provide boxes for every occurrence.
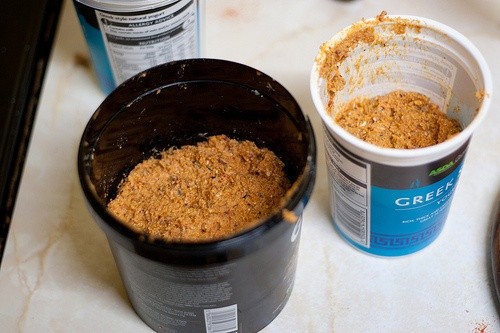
[73,0,205,94]
[77,57,317,333]
[308,14,492,257]
